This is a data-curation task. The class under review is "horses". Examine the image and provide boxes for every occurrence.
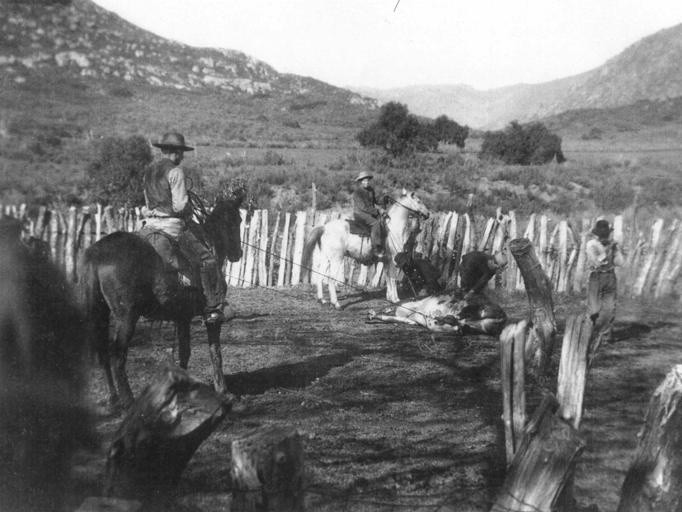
[363,284,509,339]
[76,192,256,414]
[293,185,433,309]
[1,203,85,511]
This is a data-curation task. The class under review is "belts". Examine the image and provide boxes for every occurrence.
[146,215,183,218]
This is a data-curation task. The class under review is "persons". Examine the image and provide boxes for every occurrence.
[137,132,236,324]
[353,171,391,262]
[460,251,508,300]
[394,252,442,299]
[585,219,627,344]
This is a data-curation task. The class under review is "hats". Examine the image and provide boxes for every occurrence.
[153,133,194,152]
[355,172,373,182]
[593,220,614,235]
